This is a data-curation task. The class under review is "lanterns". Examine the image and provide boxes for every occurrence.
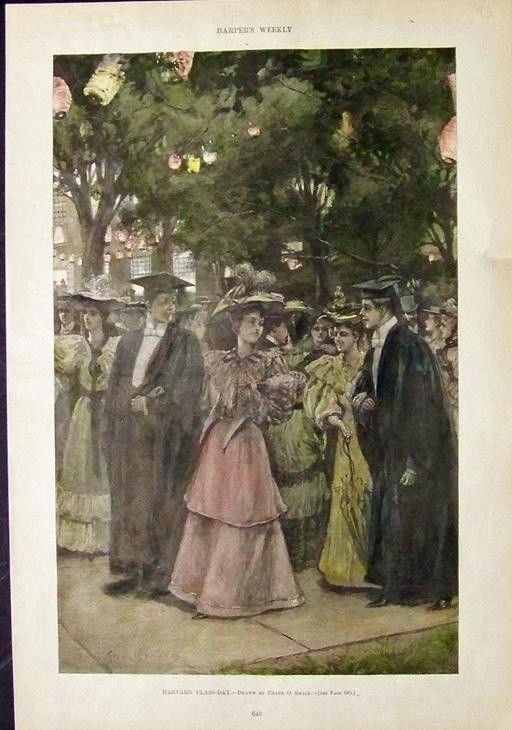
[168,154,182,168]
[203,150,216,164]
[157,52,195,81]
[51,75,74,123]
[83,52,133,109]
[436,116,457,167]
[185,156,200,174]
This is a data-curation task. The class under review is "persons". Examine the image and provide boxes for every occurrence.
[57,271,458,625]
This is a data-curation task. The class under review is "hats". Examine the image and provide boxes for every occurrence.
[422,298,458,319]
[322,278,419,321]
[210,283,313,318]
[54,291,127,309]
[128,271,195,298]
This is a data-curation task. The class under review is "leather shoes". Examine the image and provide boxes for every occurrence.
[427,597,452,611]
[192,613,208,619]
[366,598,389,607]
